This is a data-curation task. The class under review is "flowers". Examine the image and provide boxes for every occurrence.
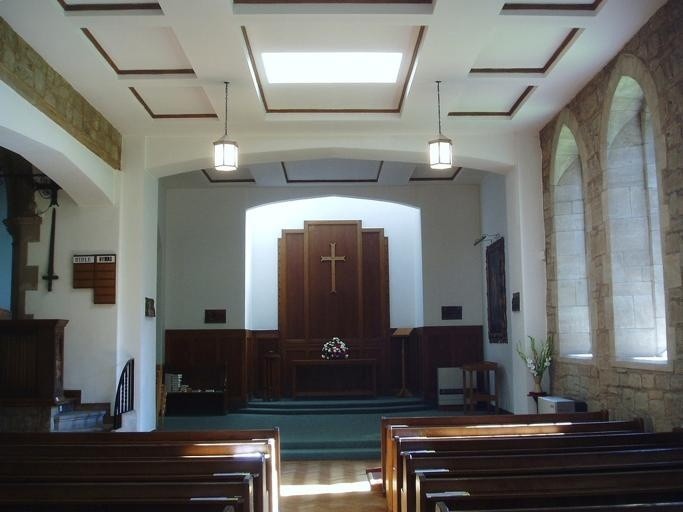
[514,333,557,375]
[320,337,348,360]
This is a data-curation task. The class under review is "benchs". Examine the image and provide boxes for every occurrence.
[0,427,280,511]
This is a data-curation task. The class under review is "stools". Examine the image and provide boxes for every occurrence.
[462,361,499,414]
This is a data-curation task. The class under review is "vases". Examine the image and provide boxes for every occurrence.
[534,375,543,393]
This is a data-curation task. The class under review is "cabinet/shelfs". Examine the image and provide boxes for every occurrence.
[0,318,69,405]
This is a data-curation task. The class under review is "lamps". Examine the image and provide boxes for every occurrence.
[428,81,451,170]
[212,82,238,173]
